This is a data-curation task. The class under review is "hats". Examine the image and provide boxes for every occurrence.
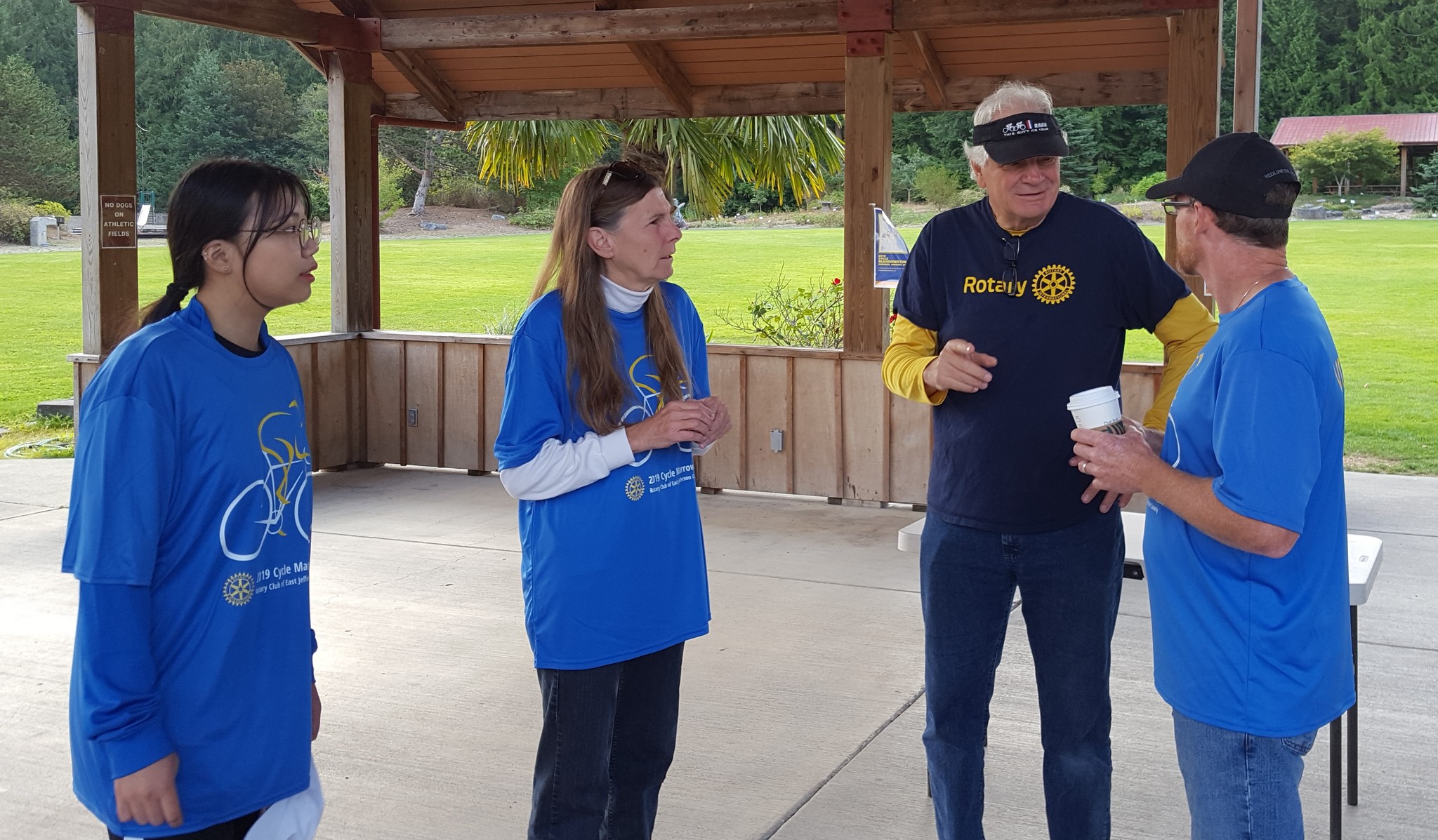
[972,112,1070,164]
[1145,132,1303,218]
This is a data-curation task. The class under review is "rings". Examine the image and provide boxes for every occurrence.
[1081,461,1093,474]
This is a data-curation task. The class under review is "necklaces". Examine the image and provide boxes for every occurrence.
[1231,265,1291,312]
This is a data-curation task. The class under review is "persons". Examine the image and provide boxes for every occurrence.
[492,161,732,840]
[61,159,323,839]
[884,77,1218,839]
[1070,132,1356,840]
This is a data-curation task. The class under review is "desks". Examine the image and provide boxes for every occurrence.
[897,511,1384,840]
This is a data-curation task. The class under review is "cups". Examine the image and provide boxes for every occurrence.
[1066,385,1126,436]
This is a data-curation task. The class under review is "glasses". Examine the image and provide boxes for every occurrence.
[230,214,324,248]
[1161,198,1191,215]
[589,158,647,214]
